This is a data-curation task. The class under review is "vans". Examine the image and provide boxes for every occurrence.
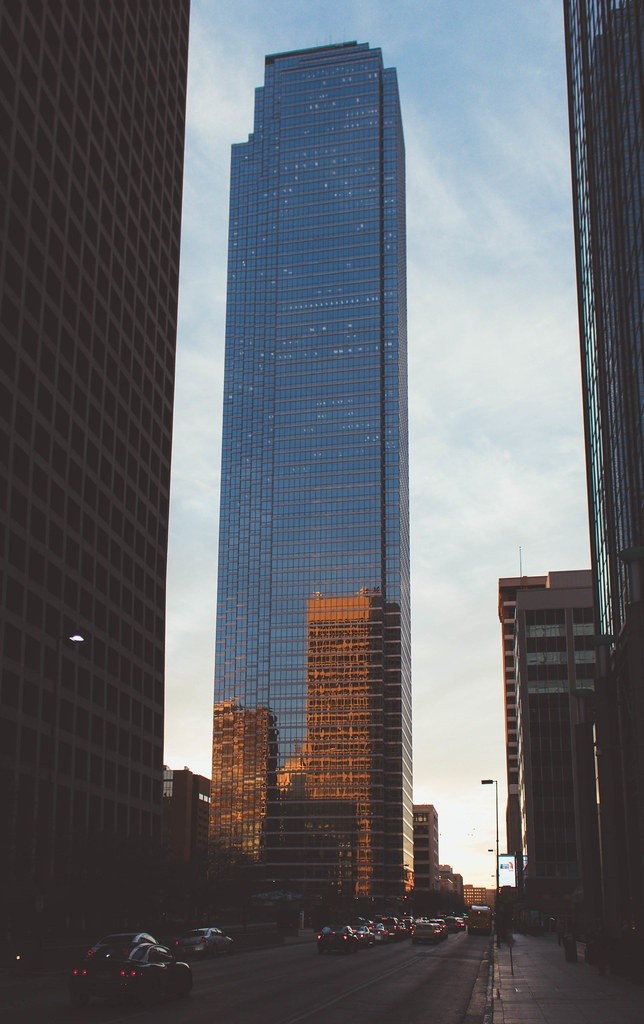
[468,910,490,935]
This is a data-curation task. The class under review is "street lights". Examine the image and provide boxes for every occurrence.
[482,780,500,948]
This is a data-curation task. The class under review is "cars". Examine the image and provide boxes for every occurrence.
[176,928,233,959]
[73,933,192,1004]
[318,926,358,954]
[429,919,448,938]
[364,912,467,944]
[412,923,442,944]
[351,925,374,947]
